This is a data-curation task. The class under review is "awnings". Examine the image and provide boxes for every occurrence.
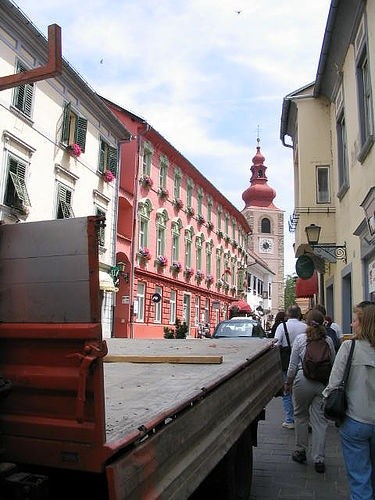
[231,300,252,314]
[294,272,318,297]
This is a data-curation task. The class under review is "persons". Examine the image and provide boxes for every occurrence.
[328,301,375,500]
[271,304,342,473]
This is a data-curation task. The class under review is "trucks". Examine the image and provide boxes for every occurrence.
[2,210,285,500]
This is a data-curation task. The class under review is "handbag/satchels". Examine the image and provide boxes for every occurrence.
[324,388,348,421]
[278,345,292,371]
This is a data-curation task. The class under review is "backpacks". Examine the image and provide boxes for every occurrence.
[325,322,340,354]
[299,339,333,383]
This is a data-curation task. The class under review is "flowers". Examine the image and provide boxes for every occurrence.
[102,169,114,182]
[68,143,82,155]
[140,176,248,292]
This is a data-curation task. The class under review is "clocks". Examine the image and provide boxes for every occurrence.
[259,239,273,254]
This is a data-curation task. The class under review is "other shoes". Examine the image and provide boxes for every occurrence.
[282,421,295,428]
[314,462,325,473]
[293,450,307,463]
[307,423,312,433]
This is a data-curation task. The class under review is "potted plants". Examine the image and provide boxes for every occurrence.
[163,325,174,338]
[175,318,189,338]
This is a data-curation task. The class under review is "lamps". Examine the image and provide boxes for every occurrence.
[117,260,130,283]
[305,223,347,264]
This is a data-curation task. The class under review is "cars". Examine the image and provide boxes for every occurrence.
[205,316,267,338]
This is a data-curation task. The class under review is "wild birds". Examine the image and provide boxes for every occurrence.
[233,8,245,14]
[100,59,103,65]
